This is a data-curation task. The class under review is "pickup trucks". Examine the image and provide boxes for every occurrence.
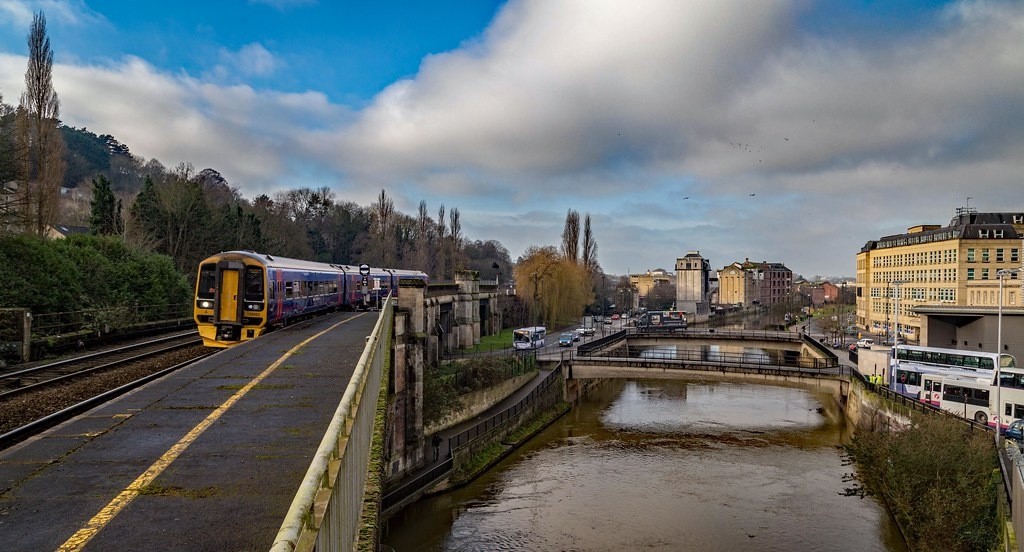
[883,337,907,345]
[849,326,859,335]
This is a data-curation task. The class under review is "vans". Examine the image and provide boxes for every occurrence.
[857,338,874,349]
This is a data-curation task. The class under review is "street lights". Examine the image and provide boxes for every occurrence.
[646,285,649,307]
[894,280,903,392]
[993,269,1011,440]
[787,293,789,304]
[621,290,623,330]
[629,290,630,318]
[808,295,810,336]
[600,276,605,337]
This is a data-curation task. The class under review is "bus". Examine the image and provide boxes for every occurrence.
[785,309,804,322]
[891,344,1024,434]
[513,326,546,350]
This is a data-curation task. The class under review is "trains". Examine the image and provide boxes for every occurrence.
[194,249,429,347]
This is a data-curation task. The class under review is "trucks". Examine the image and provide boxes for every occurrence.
[636,311,687,333]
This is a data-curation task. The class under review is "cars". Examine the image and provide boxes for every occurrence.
[605,314,630,324]
[557,325,596,347]
[1005,420,1024,443]
